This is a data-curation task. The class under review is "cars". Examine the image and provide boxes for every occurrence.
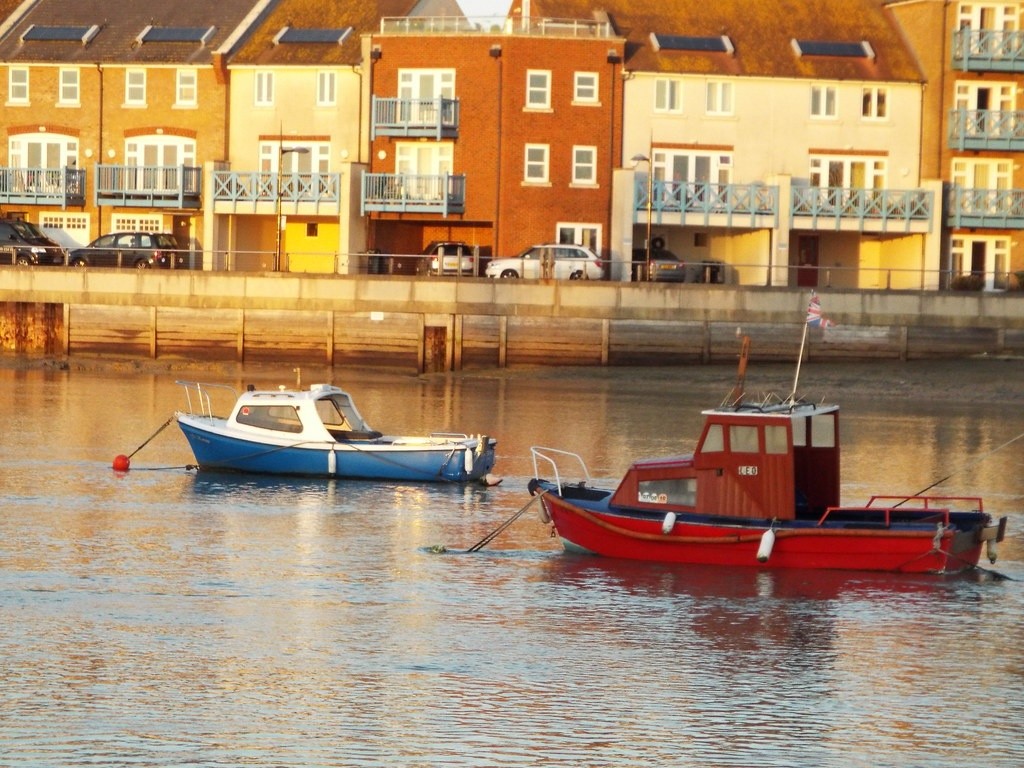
[632,248,688,283]
[417,242,474,277]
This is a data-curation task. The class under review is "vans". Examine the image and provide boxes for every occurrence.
[1,221,64,268]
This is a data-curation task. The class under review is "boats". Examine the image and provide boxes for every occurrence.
[172,379,498,484]
[529,335,1007,575]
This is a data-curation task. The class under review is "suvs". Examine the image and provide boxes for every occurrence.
[485,244,605,281]
[68,232,183,271]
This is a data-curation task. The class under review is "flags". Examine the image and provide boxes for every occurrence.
[807,296,837,330]
